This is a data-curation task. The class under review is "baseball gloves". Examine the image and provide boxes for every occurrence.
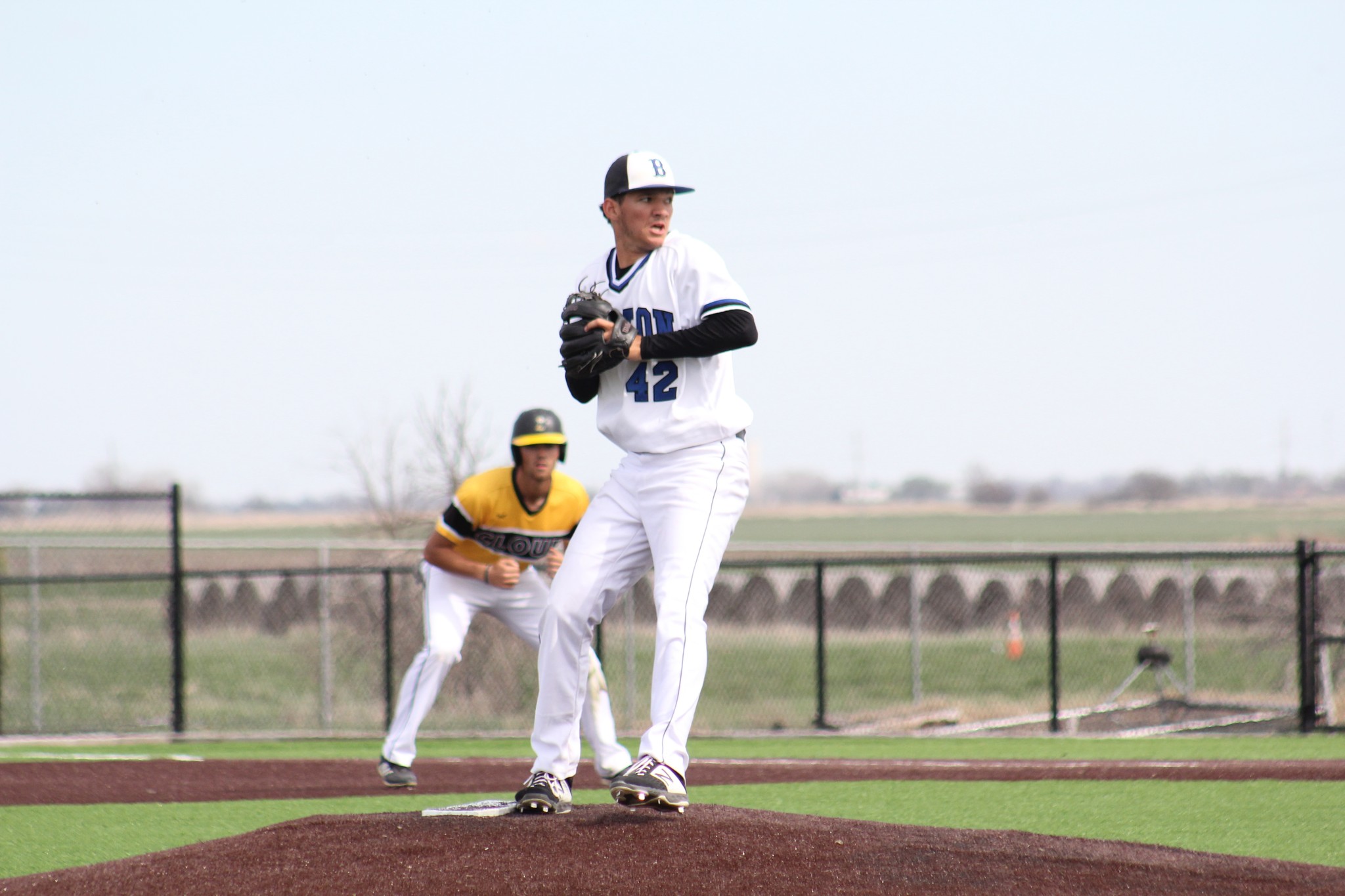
[560,293,639,374]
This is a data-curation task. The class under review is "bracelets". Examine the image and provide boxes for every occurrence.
[482,564,492,584]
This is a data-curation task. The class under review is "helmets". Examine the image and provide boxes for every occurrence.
[511,409,567,466]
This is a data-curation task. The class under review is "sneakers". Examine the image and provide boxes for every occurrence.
[610,755,689,816]
[515,770,575,814]
[375,756,417,789]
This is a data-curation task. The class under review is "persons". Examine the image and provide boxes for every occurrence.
[515,150,759,814]
[987,611,1024,659]
[1133,622,1193,704]
[378,409,635,789]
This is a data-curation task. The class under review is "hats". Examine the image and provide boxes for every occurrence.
[604,150,695,202]
